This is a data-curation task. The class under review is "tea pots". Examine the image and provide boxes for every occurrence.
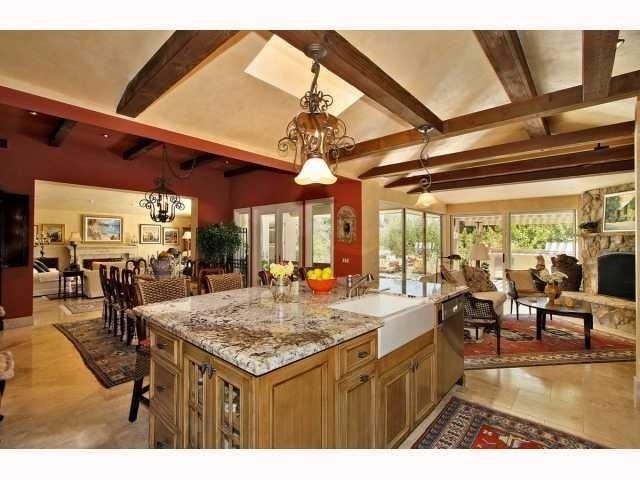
[269,273,292,303]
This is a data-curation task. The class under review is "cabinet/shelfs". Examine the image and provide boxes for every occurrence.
[376,327,437,449]
[333,330,381,450]
[146,320,338,449]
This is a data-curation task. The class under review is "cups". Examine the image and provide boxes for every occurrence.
[566,297,577,307]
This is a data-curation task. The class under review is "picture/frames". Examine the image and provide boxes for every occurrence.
[602,190,636,235]
[162,226,179,245]
[82,214,124,244]
[41,223,65,245]
[33,224,39,248]
[140,224,161,244]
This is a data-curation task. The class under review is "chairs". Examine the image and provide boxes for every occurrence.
[128,279,190,422]
[97,258,231,345]
[288,265,314,282]
[204,272,243,293]
[440,266,502,355]
[258,271,273,285]
[505,268,554,320]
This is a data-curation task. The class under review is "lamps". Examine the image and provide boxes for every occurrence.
[435,254,462,283]
[69,232,82,269]
[468,243,490,268]
[413,123,440,210]
[138,147,185,223]
[181,232,192,260]
[35,231,51,257]
[278,41,357,188]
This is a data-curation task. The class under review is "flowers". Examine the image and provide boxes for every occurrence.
[539,269,569,283]
[270,261,294,285]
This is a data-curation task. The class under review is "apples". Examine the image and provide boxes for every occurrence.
[306,267,335,280]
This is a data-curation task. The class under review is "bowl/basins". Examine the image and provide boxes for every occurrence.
[306,278,337,292]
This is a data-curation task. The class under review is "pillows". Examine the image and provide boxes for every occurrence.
[508,271,536,292]
[461,265,498,293]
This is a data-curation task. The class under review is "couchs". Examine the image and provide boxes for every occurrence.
[33,254,133,300]
[420,270,509,341]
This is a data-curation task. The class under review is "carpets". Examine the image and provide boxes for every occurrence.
[397,384,617,449]
[463,313,639,371]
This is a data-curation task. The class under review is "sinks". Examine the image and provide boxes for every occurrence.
[329,296,435,361]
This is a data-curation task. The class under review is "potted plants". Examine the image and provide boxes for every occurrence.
[579,220,598,233]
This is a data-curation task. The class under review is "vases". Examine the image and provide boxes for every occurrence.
[544,281,563,300]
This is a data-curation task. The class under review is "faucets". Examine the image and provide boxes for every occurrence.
[346,272,375,299]
